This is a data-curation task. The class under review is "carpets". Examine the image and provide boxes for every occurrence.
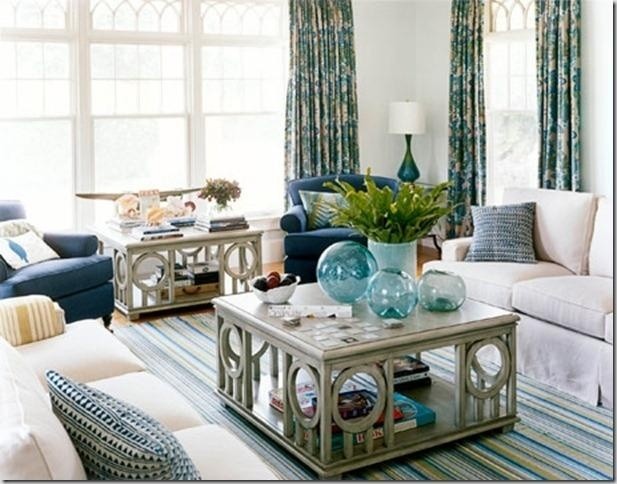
[112,314,613,480]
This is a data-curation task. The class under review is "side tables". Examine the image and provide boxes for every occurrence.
[398,182,447,259]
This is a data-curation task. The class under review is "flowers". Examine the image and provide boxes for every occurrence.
[196,178,242,202]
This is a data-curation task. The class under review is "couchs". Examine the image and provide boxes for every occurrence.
[0,295,282,480]
[421,186,613,412]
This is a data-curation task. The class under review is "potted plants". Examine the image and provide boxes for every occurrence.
[321,168,465,297]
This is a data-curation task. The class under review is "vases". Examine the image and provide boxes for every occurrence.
[211,201,233,215]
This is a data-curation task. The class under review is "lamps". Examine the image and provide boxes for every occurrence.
[388,100,428,184]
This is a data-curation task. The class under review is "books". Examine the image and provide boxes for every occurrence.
[265,352,435,455]
[128,211,250,286]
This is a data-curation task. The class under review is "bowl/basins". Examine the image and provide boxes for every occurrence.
[249,274,301,305]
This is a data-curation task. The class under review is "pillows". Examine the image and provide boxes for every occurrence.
[0,335,202,481]
[298,189,345,231]
[0,218,60,270]
[463,184,613,276]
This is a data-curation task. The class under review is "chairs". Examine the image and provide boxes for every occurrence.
[0,199,114,325]
[281,174,398,283]
